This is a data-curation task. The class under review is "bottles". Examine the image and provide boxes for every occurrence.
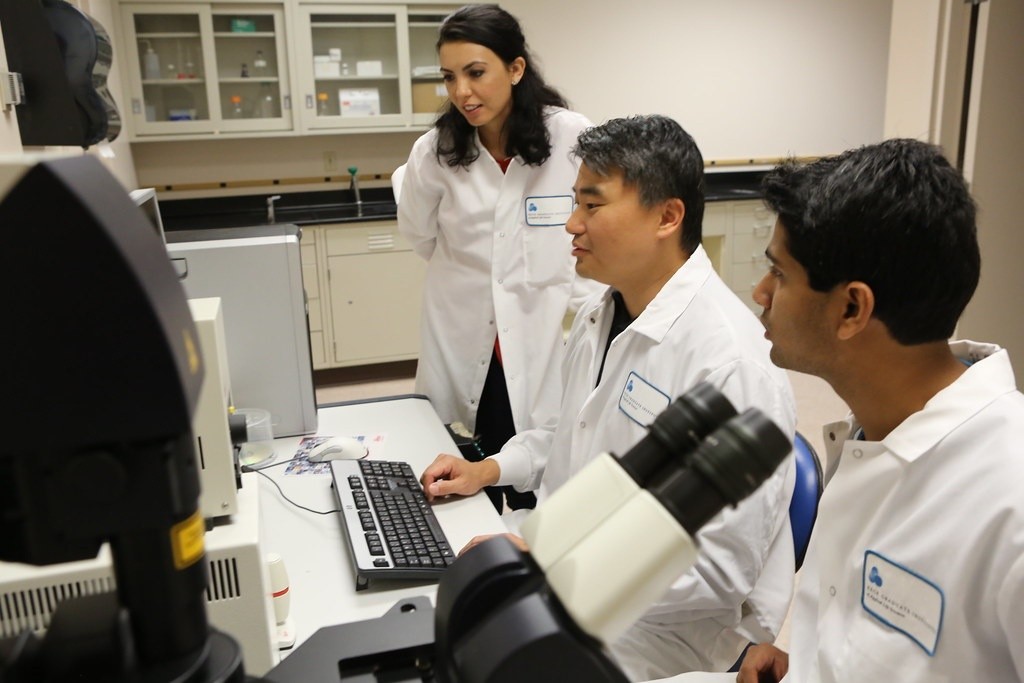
[145,48,161,79]
[231,96,243,119]
[168,63,196,79]
[262,96,275,117]
[319,94,329,116]
[254,50,266,77]
[241,63,249,78]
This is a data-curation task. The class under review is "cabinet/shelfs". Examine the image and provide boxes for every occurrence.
[113,1,499,143]
[702,200,778,319]
[299,220,424,370]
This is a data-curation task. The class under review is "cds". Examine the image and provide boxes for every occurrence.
[238,442,275,466]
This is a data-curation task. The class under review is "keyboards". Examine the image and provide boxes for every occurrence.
[329,458,456,591]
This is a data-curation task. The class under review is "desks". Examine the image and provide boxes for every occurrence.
[241,394,509,660]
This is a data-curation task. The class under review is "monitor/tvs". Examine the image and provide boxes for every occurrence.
[129,189,169,250]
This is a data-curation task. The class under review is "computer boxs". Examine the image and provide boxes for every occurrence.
[167,233,318,440]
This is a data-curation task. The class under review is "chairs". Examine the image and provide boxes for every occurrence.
[726,431,823,673]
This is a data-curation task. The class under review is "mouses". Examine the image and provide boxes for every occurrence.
[308,437,369,462]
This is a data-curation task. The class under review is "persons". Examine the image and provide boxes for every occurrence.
[419,115,797,683]
[644,138,1024,683]
[396,4,599,515]
